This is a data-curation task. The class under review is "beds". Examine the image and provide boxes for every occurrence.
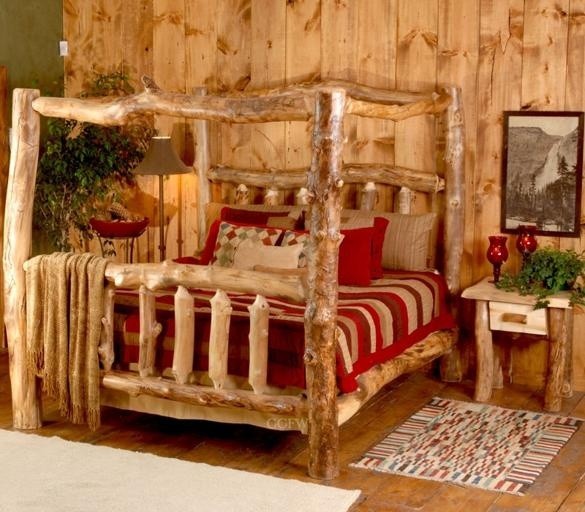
[1,82,469,479]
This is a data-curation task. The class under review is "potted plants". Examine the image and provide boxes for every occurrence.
[491,240,585,313]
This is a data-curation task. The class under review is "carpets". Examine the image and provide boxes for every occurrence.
[2,426,365,511]
[344,393,584,500]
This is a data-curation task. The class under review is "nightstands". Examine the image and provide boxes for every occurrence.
[461,273,578,414]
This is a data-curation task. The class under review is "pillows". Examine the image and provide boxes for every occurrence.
[194,207,439,287]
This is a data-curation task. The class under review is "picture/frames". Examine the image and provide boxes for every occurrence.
[499,107,585,240]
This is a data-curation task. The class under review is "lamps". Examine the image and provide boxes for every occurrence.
[127,129,194,266]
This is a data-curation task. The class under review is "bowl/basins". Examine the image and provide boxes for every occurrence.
[88,216,151,239]
